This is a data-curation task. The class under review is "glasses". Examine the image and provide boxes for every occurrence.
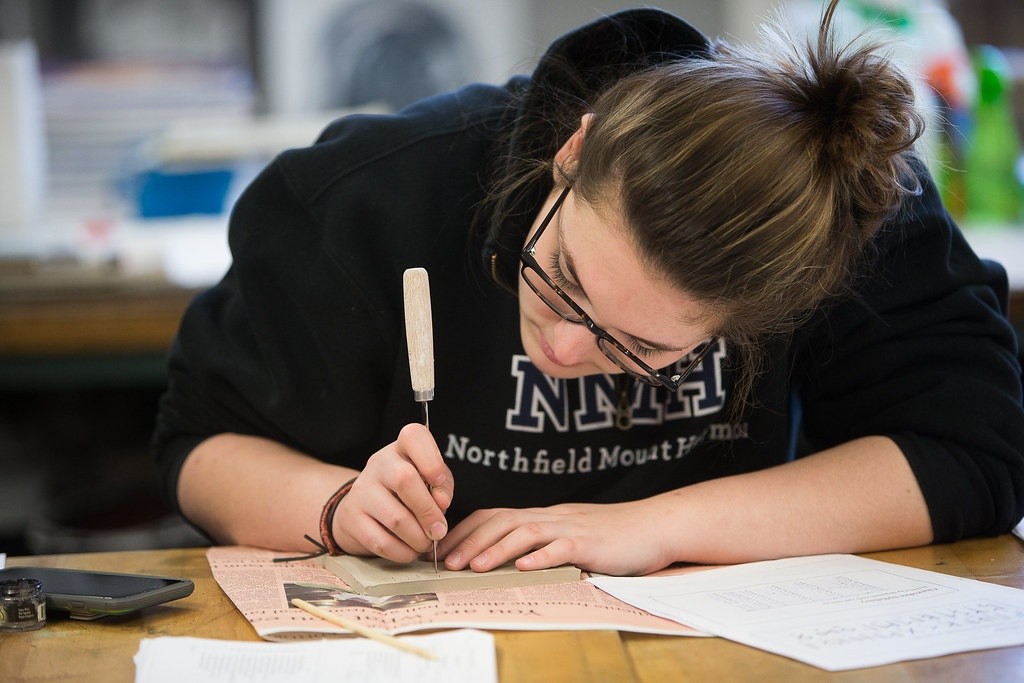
[520,159,732,392]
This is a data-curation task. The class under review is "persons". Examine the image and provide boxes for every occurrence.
[143,8,1024,578]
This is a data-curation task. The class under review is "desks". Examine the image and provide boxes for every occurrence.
[0,288,200,357]
[0,531,1024,683]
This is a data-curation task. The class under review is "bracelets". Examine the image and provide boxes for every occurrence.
[320,477,357,556]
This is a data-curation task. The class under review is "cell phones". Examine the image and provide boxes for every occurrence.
[0,566,193,616]
